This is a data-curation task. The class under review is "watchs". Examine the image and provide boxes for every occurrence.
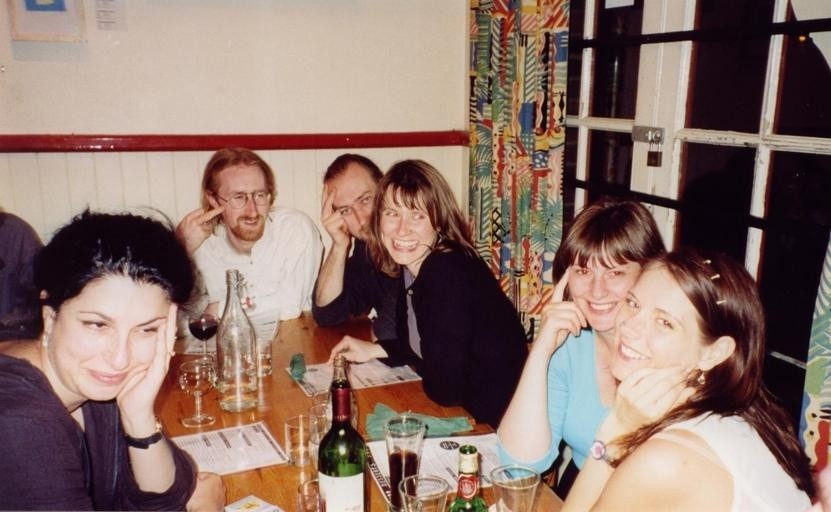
[592,439,619,469]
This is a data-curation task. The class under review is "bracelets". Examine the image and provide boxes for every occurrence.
[124,417,164,448]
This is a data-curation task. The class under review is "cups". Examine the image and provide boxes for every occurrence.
[489,464,540,512]
[286,389,357,469]
[295,478,318,511]
[382,417,449,512]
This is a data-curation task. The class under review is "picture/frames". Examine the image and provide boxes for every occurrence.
[7,0,86,42]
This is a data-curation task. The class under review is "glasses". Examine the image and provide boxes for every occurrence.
[212,190,271,210]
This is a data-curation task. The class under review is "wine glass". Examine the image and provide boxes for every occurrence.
[175,267,272,429]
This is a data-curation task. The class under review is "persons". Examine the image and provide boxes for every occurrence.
[173,142,322,322]
[328,158,529,428]
[315,151,391,319]
[494,198,667,480]
[556,249,815,510]
[3,210,228,511]
[0,209,46,339]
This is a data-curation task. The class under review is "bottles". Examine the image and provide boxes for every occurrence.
[317,356,371,512]
[449,445,490,512]
